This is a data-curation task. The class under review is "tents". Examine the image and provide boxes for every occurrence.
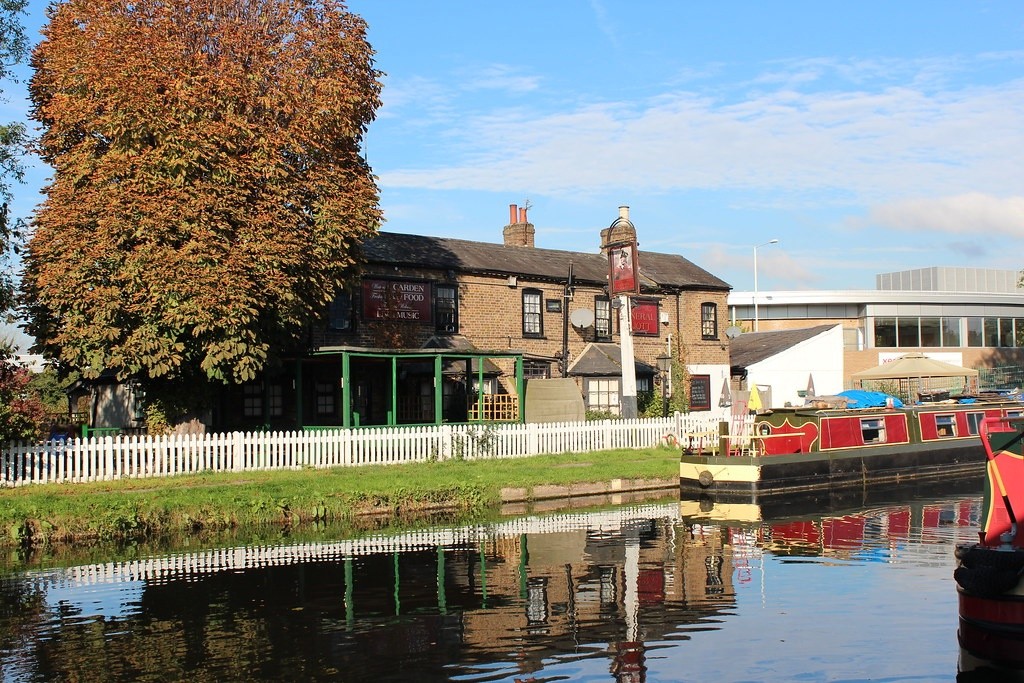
[851,352,979,403]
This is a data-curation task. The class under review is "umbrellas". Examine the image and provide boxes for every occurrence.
[747,379,763,415]
[718,378,732,408]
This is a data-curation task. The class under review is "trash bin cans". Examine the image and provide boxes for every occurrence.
[51,432,70,460]
[917,392,950,402]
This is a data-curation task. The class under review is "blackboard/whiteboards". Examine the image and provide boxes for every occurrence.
[687,374,712,412]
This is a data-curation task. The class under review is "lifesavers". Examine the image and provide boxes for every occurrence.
[661,429,680,449]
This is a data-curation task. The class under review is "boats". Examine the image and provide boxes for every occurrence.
[952,411,1024,683]
[677,389,1024,499]
